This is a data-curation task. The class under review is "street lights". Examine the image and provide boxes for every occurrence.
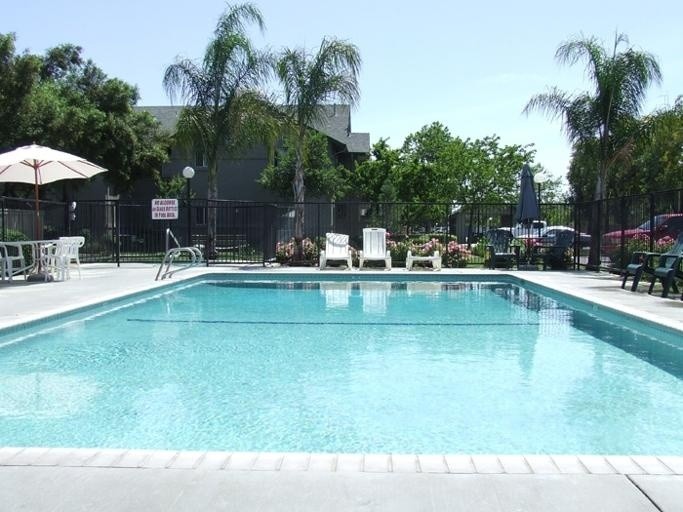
[181,166,195,262]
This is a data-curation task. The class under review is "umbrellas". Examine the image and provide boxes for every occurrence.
[515,162,541,253]
[0,140,110,274]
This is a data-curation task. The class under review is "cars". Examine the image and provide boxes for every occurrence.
[472,219,593,257]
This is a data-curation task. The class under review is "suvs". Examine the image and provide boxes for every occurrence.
[600,213,682,268]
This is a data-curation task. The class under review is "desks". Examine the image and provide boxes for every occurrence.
[512,236,553,271]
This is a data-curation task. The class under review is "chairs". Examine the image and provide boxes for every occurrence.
[648,253,683,300]
[358,228,393,272]
[319,233,353,270]
[318,281,442,315]
[621,232,683,292]
[0,236,85,283]
[485,244,515,269]
[534,230,574,271]
[406,250,442,272]
